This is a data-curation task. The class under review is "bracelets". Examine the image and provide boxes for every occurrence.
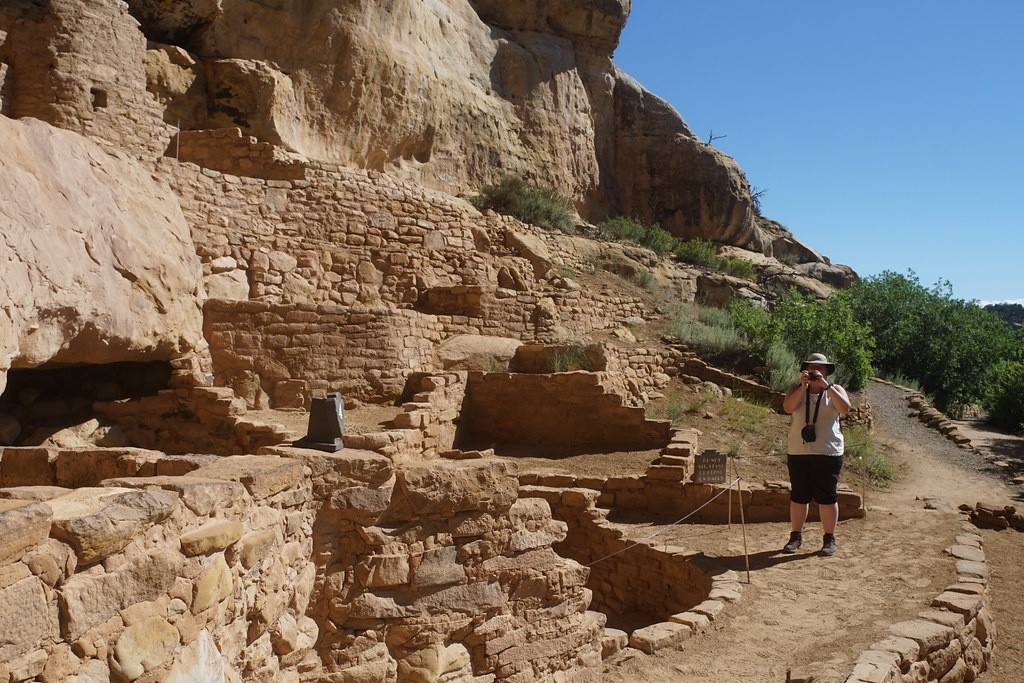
[825,384,831,389]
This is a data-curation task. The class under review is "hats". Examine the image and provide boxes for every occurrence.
[800,353,835,376]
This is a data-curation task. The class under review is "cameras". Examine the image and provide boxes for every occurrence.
[807,371,817,380]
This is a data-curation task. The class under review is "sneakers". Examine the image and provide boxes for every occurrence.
[820,536,835,557]
[783,537,802,552]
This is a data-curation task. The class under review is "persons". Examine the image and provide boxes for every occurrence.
[784,353,851,555]
